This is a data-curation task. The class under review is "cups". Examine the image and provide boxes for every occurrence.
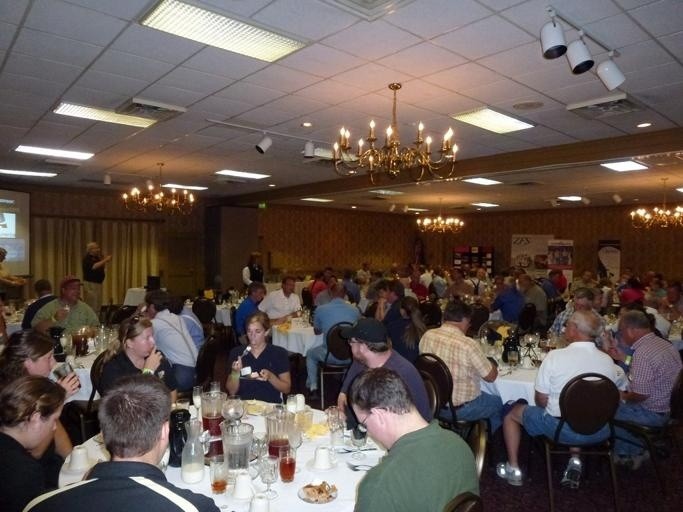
[69,445,88,471]
[174,399,191,410]
[287,394,298,412]
[218,424,255,471]
[295,394,305,411]
[279,447,297,482]
[330,418,346,447]
[210,381,222,392]
[200,391,226,437]
[266,412,296,459]
[232,473,257,500]
[105,324,121,341]
[72,327,89,359]
[313,447,338,470]
[210,456,228,494]
[519,334,540,350]
[93,325,110,355]
[53,361,82,390]
[250,494,270,512]
[17,312,24,322]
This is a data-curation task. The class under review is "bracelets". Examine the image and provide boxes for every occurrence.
[50,314,59,324]
[143,365,156,379]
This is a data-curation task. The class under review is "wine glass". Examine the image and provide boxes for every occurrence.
[260,456,279,500]
[253,432,268,471]
[60,333,67,352]
[508,350,518,376]
[350,430,367,460]
[298,410,314,442]
[221,395,244,424]
[303,309,310,328]
[479,328,492,357]
[328,406,340,434]
[296,307,302,324]
[65,333,74,356]
[193,386,202,417]
[288,423,302,473]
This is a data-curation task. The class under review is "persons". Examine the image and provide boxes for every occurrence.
[460,261,546,337]
[324,317,431,425]
[0,330,80,401]
[590,310,682,481]
[310,260,460,310]
[496,310,617,489]
[233,281,304,346]
[0,375,74,512]
[22,373,221,512]
[242,252,264,288]
[546,269,683,343]
[169,294,205,353]
[31,277,101,339]
[83,242,113,319]
[305,283,362,396]
[141,289,200,393]
[418,300,504,475]
[257,275,302,319]
[0,247,27,339]
[97,315,184,402]
[226,310,293,405]
[21,279,60,329]
[348,366,480,512]
[386,296,428,366]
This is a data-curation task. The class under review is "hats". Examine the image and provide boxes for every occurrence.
[552,277,568,290]
[60,275,85,289]
[619,287,645,306]
[340,317,389,344]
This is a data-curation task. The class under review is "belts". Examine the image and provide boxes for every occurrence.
[444,401,473,411]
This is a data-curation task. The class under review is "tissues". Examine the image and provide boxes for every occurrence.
[285,393,314,434]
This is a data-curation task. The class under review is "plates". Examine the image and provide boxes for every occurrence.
[298,481,340,505]
[245,400,272,415]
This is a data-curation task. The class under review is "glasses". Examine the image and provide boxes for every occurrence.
[346,337,367,345]
[126,313,145,337]
[356,408,387,432]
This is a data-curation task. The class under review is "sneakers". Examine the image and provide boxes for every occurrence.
[560,455,582,493]
[496,462,524,487]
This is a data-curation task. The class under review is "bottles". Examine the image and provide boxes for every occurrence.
[181,419,205,485]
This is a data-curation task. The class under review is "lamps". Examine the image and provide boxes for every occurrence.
[205,117,334,157]
[415,207,464,235]
[539,4,627,91]
[630,177,683,232]
[334,84,459,186]
[123,163,195,216]
[103,173,110,184]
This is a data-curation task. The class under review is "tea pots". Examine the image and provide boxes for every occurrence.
[45,326,67,362]
[503,337,520,365]
